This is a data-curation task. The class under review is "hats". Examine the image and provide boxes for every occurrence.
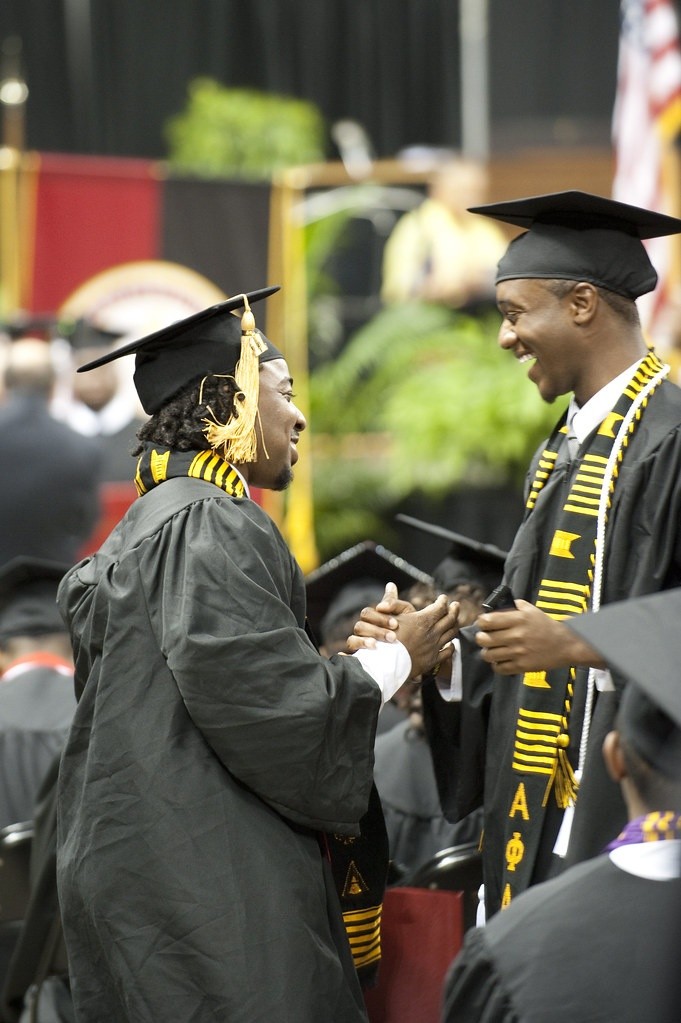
[59,319,124,350]
[542,588,681,810]
[302,541,431,652]
[77,285,283,464]
[0,554,77,633]
[468,189,681,302]
[5,318,55,343]
[377,515,509,597]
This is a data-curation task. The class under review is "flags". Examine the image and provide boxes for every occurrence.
[610,0,681,363]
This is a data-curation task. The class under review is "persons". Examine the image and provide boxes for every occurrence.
[57,286,460,1023]
[346,162,681,920]
[0,316,509,1023]
[440,589,681,1023]
